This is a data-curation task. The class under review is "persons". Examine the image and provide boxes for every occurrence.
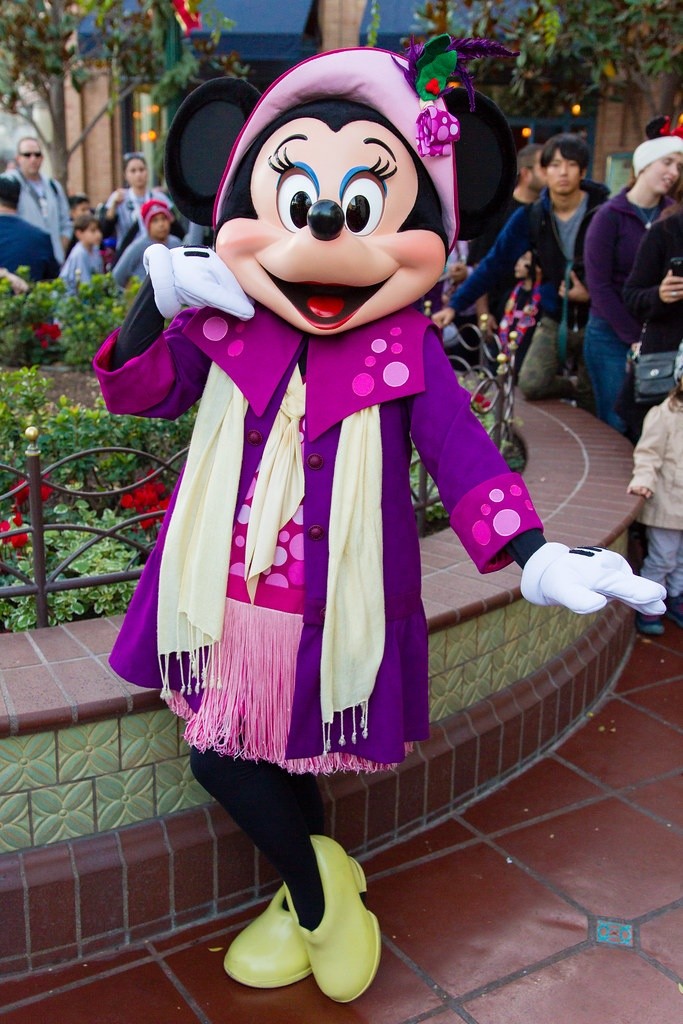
[426,113,683,445]
[626,342,683,634]
[0,136,212,324]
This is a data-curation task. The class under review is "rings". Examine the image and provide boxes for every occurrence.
[672,291,677,297]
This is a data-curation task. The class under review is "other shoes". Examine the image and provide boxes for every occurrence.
[667,604,683,627]
[635,608,664,636]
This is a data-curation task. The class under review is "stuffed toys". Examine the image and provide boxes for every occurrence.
[94,29,666,1001]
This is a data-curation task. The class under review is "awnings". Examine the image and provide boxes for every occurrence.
[76,0,314,62]
[358,0,607,60]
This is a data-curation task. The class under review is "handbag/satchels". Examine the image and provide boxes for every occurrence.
[633,350,678,404]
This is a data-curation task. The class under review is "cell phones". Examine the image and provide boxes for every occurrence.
[671,257,683,277]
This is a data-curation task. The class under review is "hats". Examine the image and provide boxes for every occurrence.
[141,198,174,233]
[672,338,683,382]
[632,136,683,177]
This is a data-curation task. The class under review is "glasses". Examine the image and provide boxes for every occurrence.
[18,152,43,159]
